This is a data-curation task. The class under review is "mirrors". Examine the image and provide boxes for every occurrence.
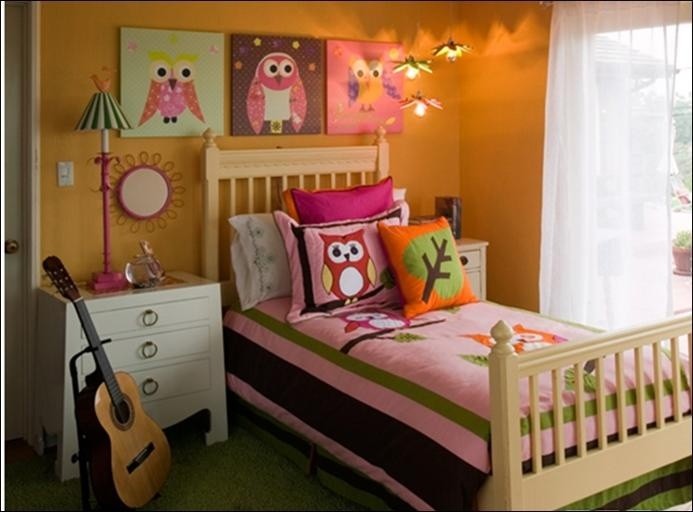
[118,165,169,218]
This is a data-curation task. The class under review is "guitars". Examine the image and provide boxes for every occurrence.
[43,255,174,510]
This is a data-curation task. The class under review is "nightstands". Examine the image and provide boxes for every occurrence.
[30,269,230,484]
[454,236,489,302]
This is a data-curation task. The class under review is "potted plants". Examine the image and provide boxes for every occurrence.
[670,230,691,277]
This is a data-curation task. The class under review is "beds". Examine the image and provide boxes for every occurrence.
[197,125,692,512]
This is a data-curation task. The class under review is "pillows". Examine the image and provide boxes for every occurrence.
[226,175,482,326]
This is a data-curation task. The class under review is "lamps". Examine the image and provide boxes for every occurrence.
[389,2,474,118]
[71,73,133,292]
